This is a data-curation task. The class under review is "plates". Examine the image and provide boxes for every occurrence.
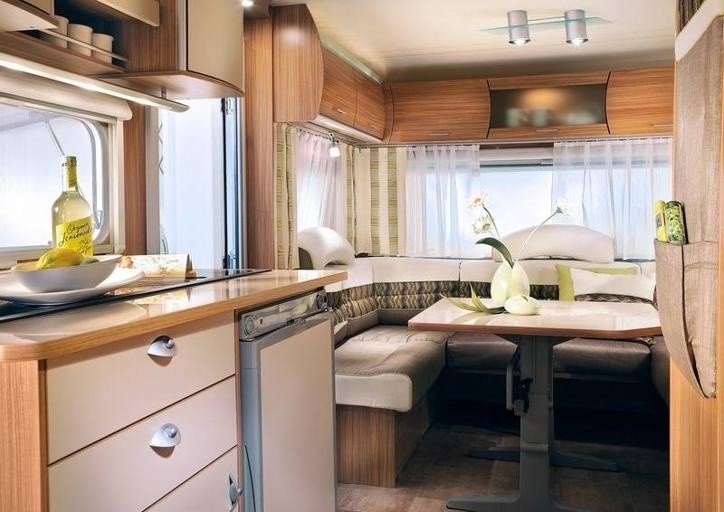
[0,265,146,308]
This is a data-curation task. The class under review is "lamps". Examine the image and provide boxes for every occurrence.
[508,9,588,47]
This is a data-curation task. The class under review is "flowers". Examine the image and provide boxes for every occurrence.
[464,190,570,267]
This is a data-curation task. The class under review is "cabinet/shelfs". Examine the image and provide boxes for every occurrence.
[384,67,673,148]
[320,48,384,140]
[44,309,241,512]
[113,0,244,102]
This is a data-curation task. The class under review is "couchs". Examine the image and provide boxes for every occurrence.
[322,256,672,485]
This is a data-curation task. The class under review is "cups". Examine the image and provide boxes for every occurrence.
[38,14,115,66]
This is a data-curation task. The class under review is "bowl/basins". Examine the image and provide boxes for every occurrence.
[9,252,123,294]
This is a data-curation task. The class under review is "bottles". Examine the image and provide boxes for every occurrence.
[51,155,93,260]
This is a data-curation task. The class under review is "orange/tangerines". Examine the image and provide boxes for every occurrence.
[80,257,99,265]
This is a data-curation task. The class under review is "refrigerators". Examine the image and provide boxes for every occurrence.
[236,285,338,510]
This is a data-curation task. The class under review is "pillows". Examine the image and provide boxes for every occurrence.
[551,264,657,305]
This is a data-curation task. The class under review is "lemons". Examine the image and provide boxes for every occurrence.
[37,247,83,269]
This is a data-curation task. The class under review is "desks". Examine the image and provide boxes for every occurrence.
[405,297,663,511]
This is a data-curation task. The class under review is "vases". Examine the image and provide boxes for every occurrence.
[491,258,531,307]
